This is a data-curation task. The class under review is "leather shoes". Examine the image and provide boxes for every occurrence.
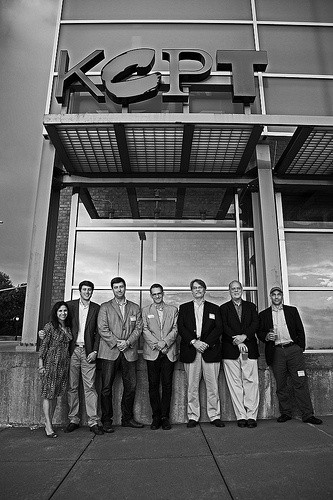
[187,420,199,428]
[103,422,114,433]
[247,419,257,428]
[210,419,225,427]
[89,424,104,435]
[64,423,79,433]
[237,419,246,427]
[302,416,323,425]
[277,414,292,422]
[121,419,144,428]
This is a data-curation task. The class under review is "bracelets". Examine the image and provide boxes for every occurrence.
[39,366,44,371]
[190,339,196,345]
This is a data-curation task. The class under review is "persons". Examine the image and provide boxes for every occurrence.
[177,279,226,428]
[97,277,145,433]
[38,301,71,439]
[38,281,105,436]
[141,284,180,429]
[258,287,323,424]
[219,280,259,429]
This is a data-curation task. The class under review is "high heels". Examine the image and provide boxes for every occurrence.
[44,428,58,438]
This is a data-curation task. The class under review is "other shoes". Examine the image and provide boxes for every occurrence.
[151,421,161,430]
[161,420,172,430]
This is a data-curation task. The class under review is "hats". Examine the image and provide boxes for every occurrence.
[270,287,283,294]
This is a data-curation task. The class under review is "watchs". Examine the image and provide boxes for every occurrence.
[94,351,98,354]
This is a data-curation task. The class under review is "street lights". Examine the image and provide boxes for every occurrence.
[138,231,147,310]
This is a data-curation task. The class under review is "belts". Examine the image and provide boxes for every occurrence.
[75,344,85,348]
[275,342,295,348]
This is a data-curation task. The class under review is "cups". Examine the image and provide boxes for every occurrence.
[242,349,248,359]
[270,328,278,340]
